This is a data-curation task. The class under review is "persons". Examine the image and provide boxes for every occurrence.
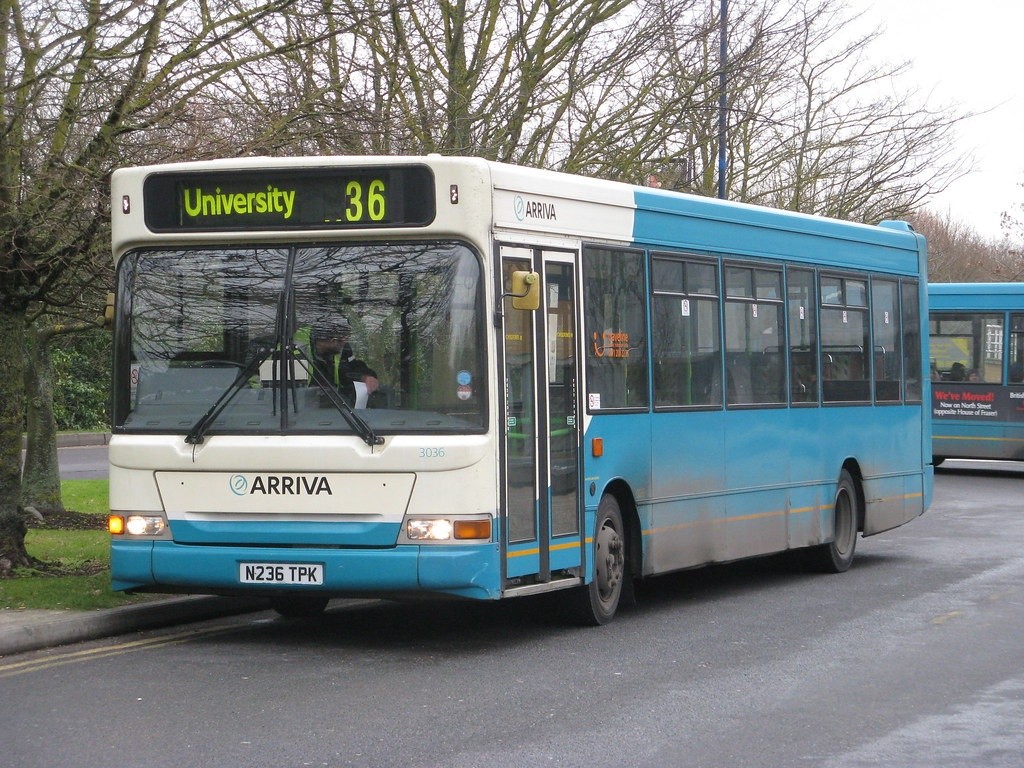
[297,313,380,395]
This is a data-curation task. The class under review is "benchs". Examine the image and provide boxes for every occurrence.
[762,344,865,381]
[762,351,833,402]
[791,345,886,381]
[655,351,721,405]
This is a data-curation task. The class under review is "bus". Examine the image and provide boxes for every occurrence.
[752,279,1024,468]
[100,151,935,629]
